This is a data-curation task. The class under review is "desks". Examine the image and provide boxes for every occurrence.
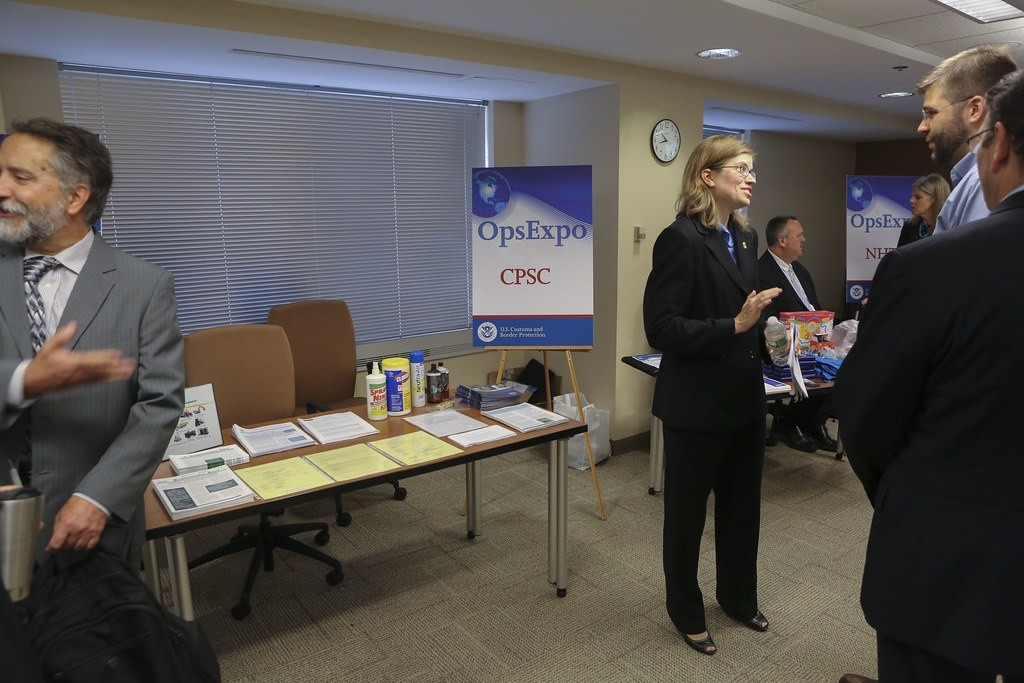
[621,346,844,494]
[144,386,588,625]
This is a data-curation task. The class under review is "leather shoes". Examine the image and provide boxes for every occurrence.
[771,415,818,451]
[800,423,838,451]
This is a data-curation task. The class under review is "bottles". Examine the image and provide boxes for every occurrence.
[437,363,449,401]
[426,363,442,404]
[410,350,425,408]
[764,316,792,366]
[381,357,411,416]
[366,362,388,420]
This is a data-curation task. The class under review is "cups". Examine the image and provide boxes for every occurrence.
[0,488,44,602]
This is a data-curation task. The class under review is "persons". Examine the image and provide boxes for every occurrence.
[897,172,950,248]
[756,213,836,455]
[0,119,185,624]
[916,44,1017,236]
[833,70,1024,683]
[643,133,783,654]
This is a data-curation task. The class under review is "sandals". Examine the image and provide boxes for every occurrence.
[741,611,769,631]
[683,629,717,655]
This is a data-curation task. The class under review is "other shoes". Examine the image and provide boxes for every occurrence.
[839,674,878,683]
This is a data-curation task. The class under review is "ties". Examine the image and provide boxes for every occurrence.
[788,268,813,312]
[22,255,60,357]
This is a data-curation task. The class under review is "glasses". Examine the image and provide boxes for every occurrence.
[965,126,1014,152]
[721,164,758,178]
[921,95,974,121]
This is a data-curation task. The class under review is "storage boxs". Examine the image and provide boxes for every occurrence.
[779,310,834,355]
[488,367,561,410]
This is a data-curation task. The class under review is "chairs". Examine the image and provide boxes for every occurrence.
[267,300,409,526]
[180,322,345,621]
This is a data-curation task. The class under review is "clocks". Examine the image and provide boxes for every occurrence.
[650,118,682,164]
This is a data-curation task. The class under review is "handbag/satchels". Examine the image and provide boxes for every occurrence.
[553,392,612,471]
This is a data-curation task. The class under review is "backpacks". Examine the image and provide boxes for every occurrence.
[20,547,221,683]
